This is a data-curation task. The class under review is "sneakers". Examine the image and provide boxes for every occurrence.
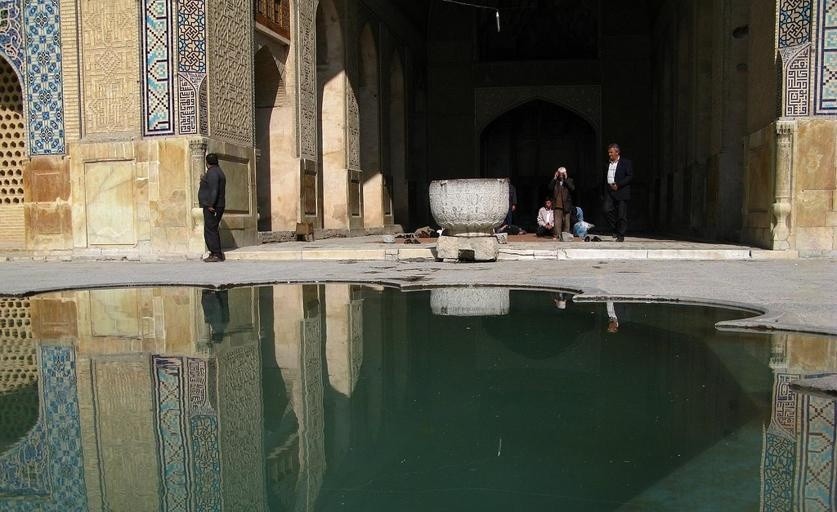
[203,253,225,262]
[613,233,623,242]
[585,235,599,242]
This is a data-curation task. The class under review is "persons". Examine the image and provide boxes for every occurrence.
[198,151,226,262]
[507,176,518,224]
[201,289,230,344]
[598,142,634,242]
[549,166,575,240]
[549,292,573,312]
[535,195,555,237]
[495,223,528,235]
[601,302,626,334]
